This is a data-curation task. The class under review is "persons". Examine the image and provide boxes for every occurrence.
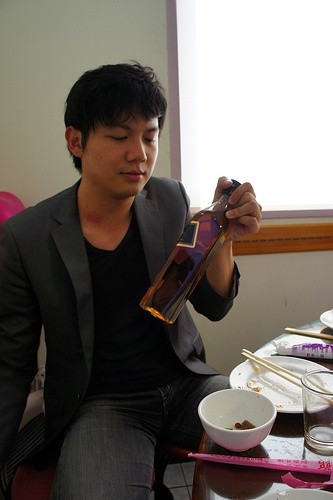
[0,64,261,500]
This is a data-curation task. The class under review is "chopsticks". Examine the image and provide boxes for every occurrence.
[285,327,333,340]
[241,348,333,404]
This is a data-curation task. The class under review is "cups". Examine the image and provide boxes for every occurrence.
[302,370,333,451]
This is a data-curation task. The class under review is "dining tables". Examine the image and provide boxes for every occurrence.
[193,320,333,500]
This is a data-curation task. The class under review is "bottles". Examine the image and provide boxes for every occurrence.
[139,179,241,324]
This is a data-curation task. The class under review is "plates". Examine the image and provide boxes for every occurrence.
[228,356,332,413]
[320,310,333,329]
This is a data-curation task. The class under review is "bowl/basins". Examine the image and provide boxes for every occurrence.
[198,389,276,452]
